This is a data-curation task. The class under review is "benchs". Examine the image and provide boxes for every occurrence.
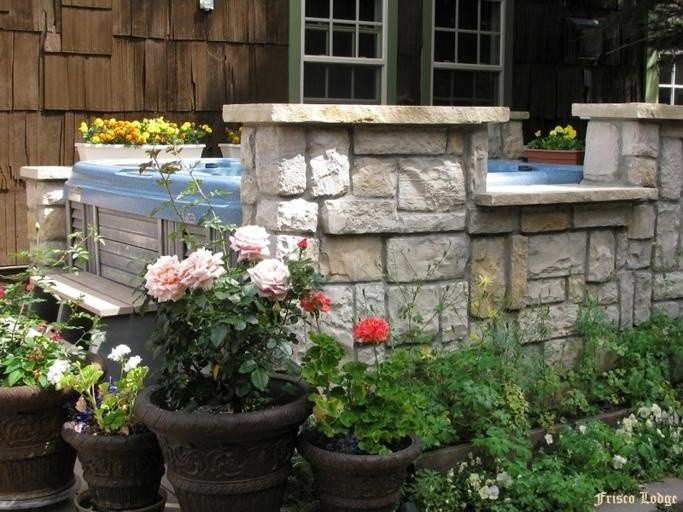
[25,271,167,383]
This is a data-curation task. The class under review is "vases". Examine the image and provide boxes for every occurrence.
[300,432,421,512]
[522,148,585,165]
[218,144,241,158]
[0,383,80,501]
[73,141,207,163]
[132,372,319,512]
[59,419,167,512]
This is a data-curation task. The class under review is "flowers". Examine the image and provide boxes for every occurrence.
[124,144,329,415]
[527,124,583,150]
[225,126,242,144]
[0,217,105,388]
[46,343,150,433]
[77,115,213,149]
[300,292,429,455]
